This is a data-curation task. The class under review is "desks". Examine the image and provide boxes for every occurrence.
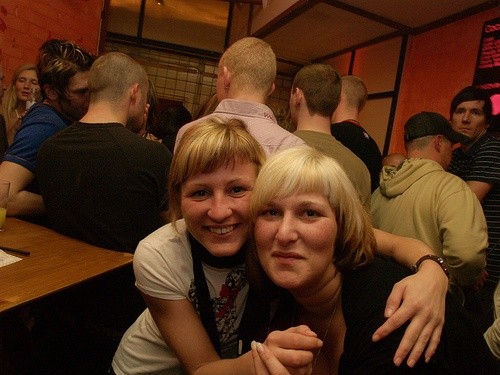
[0,215,135,317]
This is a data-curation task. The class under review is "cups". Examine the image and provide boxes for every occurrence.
[0,180,11,231]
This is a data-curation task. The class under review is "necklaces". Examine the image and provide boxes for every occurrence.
[290,307,336,368]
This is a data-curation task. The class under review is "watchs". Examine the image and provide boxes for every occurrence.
[412,254,449,278]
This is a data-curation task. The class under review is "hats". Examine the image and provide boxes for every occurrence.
[404,112,470,145]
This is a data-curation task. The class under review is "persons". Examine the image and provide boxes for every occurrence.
[109,117,448,375]
[172,36,500,325]
[246,146,500,375]
[0,39,193,375]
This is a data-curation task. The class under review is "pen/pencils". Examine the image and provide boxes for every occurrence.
[1,245,30,255]
